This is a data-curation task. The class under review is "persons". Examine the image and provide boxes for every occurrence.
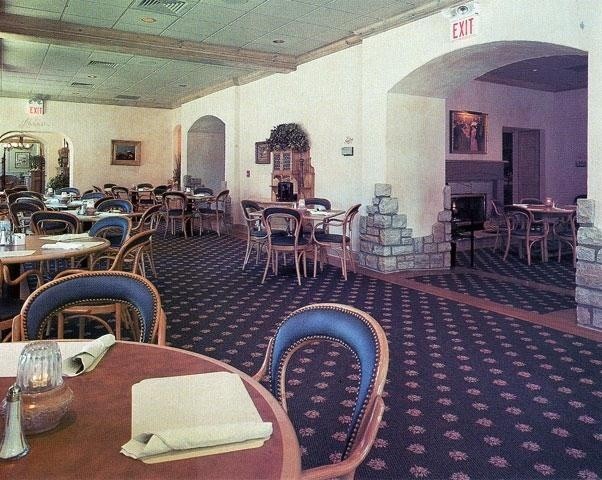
[453,117,484,152]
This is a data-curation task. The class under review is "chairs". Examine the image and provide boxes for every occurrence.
[489,197,582,266]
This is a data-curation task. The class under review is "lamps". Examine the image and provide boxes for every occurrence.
[440,0,479,22]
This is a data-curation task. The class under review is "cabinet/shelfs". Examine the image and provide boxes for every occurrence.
[30,171,44,191]
[269,147,314,206]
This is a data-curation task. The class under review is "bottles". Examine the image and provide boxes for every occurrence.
[1,386,30,461]
[1,227,8,246]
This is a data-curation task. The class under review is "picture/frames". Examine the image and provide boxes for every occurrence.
[15,152,31,168]
[110,139,141,166]
[450,110,488,155]
[255,142,270,163]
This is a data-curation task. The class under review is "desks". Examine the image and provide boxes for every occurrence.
[451,218,476,270]
